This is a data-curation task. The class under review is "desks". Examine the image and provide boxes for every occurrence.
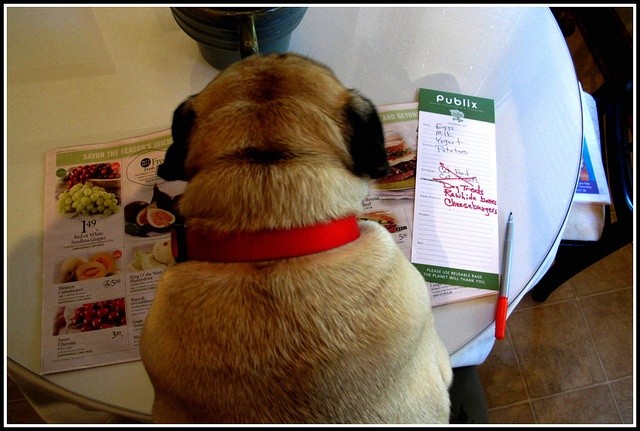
[6,7,583,422]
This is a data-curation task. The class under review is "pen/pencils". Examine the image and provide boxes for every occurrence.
[494,211,514,342]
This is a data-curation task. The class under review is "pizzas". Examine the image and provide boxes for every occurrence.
[357,213,395,233]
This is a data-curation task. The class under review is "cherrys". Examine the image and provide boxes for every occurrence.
[70,298,126,331]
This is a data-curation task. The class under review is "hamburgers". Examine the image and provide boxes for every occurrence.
[372,131,418,191]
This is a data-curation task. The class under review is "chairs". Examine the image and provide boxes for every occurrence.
[531,72,632,303]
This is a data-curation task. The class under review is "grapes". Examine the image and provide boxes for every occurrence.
[54,162,121,218]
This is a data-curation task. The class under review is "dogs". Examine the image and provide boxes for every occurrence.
[140,52,455,425]
